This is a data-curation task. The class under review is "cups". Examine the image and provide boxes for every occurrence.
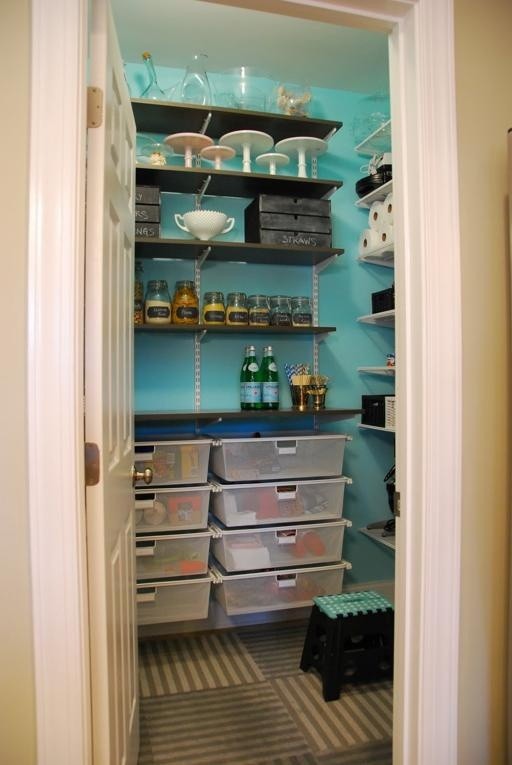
[289,384,310,408]
[173,210,235,241]
[312,385,328,407]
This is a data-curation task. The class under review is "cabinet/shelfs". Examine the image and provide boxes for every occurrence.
[353,114,399,553]
[128,98,366,432]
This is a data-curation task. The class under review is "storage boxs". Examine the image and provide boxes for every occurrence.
[132,429,353,625]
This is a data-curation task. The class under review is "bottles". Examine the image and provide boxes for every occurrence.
[277,76,312,118]
[180,52,213,106]
[134,260,144,326]
[241,344,279,408]
[144,279,312,329]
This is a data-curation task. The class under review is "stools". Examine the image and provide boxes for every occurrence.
[298,589,395,704]
